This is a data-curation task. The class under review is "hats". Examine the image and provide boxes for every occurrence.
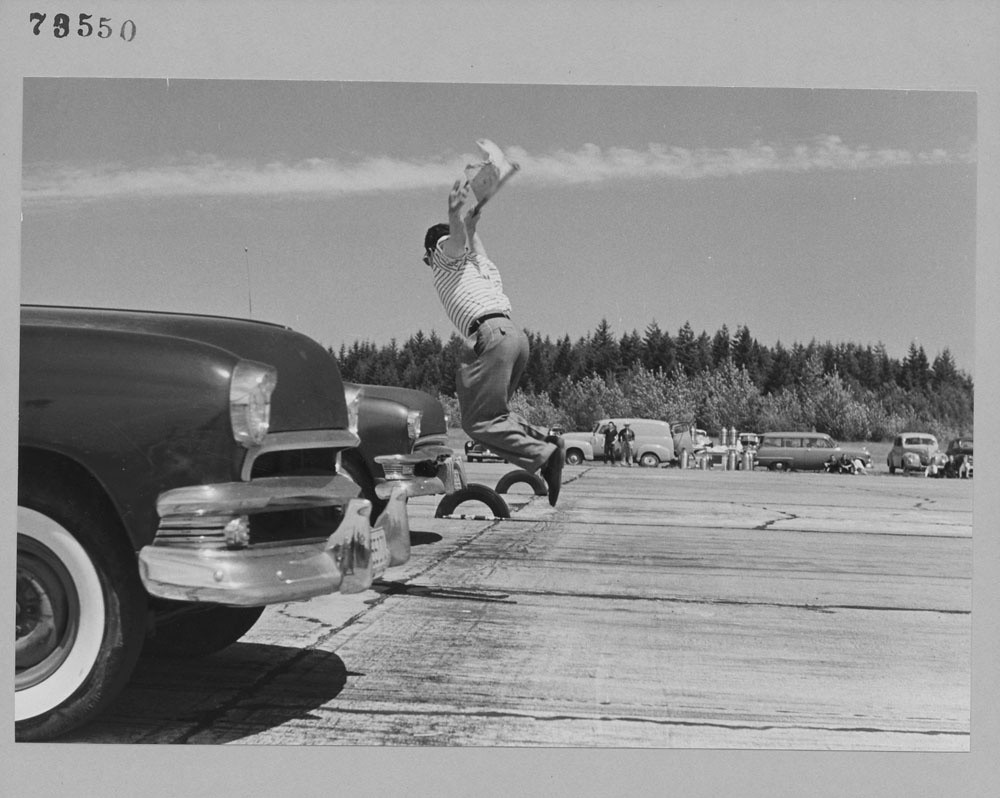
[623,422,630,426]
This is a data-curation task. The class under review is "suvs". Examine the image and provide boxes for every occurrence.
[559,418,675,465]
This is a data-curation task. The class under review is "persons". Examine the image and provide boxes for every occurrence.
[925,458,938,479]
[824,456,837,473]
[422,180,565,507]
[603,422,618,464]
[838,454,854,474]
[959,457,970,479]
[943,455,957,479]
[853,456,864,473]
[618,423,635,467]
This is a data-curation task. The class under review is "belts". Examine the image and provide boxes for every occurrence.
[471,314,510,335]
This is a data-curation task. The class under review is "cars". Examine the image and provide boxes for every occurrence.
[548,425,565,438]
[938,438,973,479]
[886,433,948,474]
[14,300,377,740]
[465,440,509,465]
[757,432,872,472]
[345,383,468,566]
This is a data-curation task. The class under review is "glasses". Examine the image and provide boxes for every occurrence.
[423,248,430,265]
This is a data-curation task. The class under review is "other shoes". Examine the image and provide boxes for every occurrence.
[545,435,567,448]
[540,446,566,507]
